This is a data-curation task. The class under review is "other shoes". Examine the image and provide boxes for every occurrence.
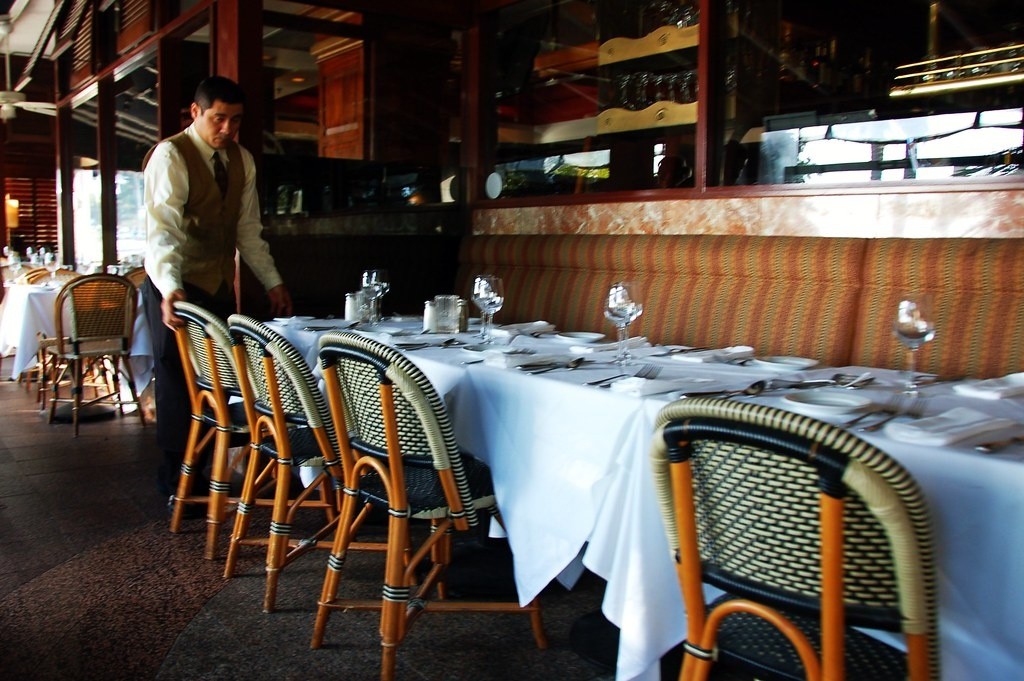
[158,468,210,519]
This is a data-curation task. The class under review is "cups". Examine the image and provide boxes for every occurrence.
[131,254,143,268]
[436,295,459,333]
[62,265,74,271]
[355,291,378,330]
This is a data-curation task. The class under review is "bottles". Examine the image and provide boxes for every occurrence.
[722,0,880,114]
[344,269,469,334]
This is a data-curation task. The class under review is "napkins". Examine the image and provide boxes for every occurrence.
[884,405,1016,447]
[611,377,713,398]
[569,336,650,354]
[488,321,555,338]
[483,352,567,369]
[951,372,1024,401]
[673,346,752,362]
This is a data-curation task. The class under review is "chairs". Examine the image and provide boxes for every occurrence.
[0,257,149,436]
[223,313,415,612]
[166,301,338,562]
[648,397,942,681]
[308,332,548,681]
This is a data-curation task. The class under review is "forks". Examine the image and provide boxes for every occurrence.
[791,373,860,388]
[835,390,928,431]
[530,331,541,338]
[582,363,663,387]
[460,347,537,365]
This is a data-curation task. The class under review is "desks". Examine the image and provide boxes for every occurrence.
[0,284,152,427]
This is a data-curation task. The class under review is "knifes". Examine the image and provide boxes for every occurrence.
[514,360,596,369]
[915,371,966,386]
[651,343,707,357]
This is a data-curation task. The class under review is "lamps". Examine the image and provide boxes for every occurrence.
[6,193,18,248]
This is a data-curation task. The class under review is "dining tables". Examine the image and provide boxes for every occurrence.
[227,317,1024,681]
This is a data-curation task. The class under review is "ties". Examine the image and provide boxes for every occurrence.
[212,151,230,198]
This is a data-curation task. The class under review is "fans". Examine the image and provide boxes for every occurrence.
[0,34,58,123]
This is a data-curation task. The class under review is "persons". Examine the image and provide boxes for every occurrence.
[143,76,292,519]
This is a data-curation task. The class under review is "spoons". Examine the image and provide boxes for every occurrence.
[527,357,584,376]
[768,378,875,387]
[399,338,455,352]
[307,320,361,332]
[722,380,766,401]
[304,322,455,352]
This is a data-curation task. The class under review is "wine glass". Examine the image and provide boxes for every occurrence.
[1,246,61,287]
[472,274,504,345]
[612,71,696,107]
[893,299,936,386]
[603,280,643,366]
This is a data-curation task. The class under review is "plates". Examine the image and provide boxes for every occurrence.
[274,316,404,336]
[783,390,872,415]
[753,355,820,369]
[389,312,424,322]
[30,284,56,291]
[468,316,481,325]
[555,332,605,343]
